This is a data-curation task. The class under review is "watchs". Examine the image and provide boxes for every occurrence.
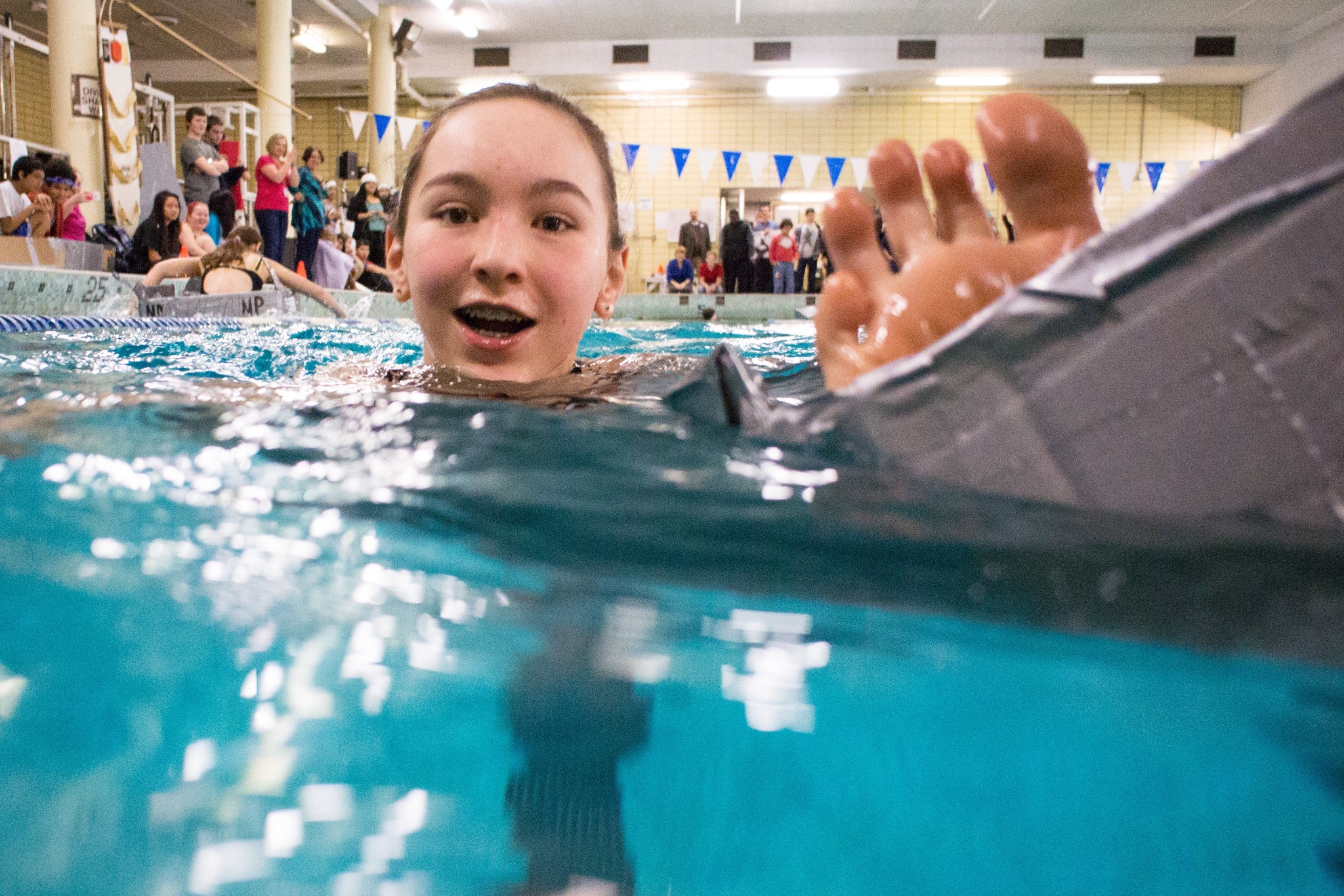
[207,157,213,163]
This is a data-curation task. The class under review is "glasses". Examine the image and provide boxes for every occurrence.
[45,176,74,186]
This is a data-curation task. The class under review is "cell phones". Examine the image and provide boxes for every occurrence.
[81,192,100,203]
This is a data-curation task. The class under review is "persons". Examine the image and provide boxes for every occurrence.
[140,226,348,321]
[1,78,1107,476]
[180,107,229,211]
[203,189,234,246]
[179,201,217,256]
[1000,205,1015,244]
[212,131,250,226]
[202,114,235,237]
[253,133,300,264]
[310,170,407,291]
[664,201,903,294]
[286,146,329,282]
[128,191,181,273]
[1,148,103,243]
